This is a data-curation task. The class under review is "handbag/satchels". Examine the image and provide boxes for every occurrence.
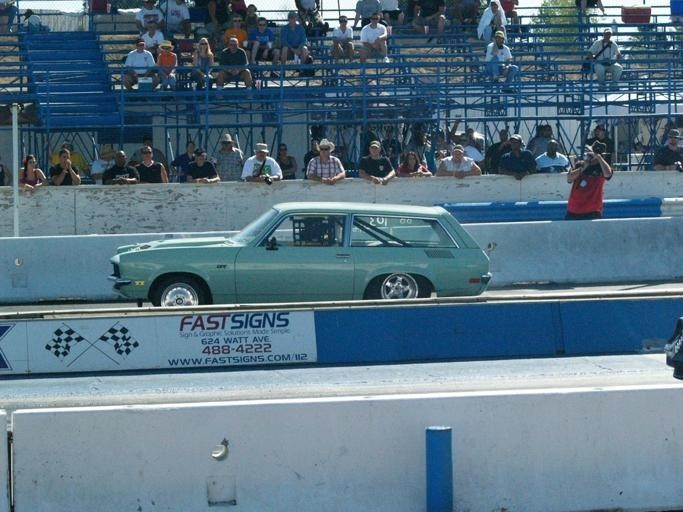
[584,55,596,70]
[306,56,315,75]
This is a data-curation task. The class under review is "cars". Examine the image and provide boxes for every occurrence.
[109,201,491,308]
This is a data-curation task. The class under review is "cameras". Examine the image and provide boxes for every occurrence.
[674,161,683,172]
[265,176,272,185]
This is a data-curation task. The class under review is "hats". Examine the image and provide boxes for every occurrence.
[603,28,612,34]
[219,133,236,144]
[667,129,682,139]
[494,31,504,39]
[509,133,522,143]
[158,40,175,51]
[315,138,335,153]
[453,145,465,153]
[586,140,609,156]
[370,140,381,149]
[255,143,270,154]
[137,38,145,46]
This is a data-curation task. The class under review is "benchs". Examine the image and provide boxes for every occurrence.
[0,1,682,109]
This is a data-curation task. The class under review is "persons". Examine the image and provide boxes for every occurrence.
[121,2,325,90]
[333,16,355,64]
[586,29,625,92]
[575,1,608,16]
[1,1,18,33]
[353,0,519,44]
[565,140,614,219]
[19,123,682,193]
[357,12,389,63]
[485,30,519,84]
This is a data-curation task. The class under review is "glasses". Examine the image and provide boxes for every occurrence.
[200,42,206,45]
[233,19,242,23]
[143,150,152,155]
